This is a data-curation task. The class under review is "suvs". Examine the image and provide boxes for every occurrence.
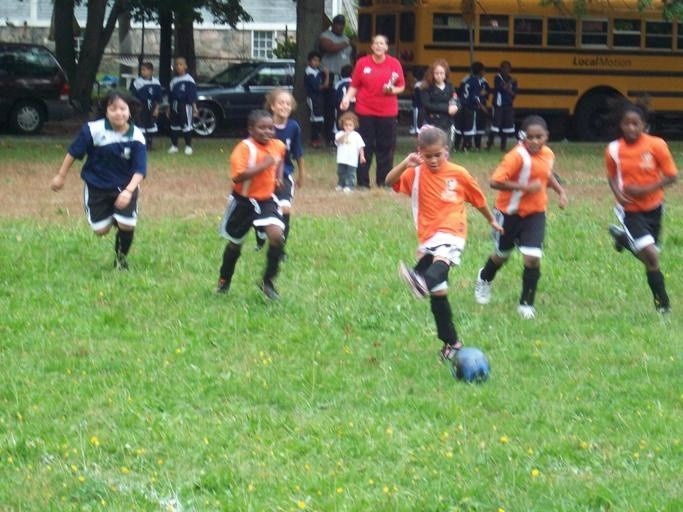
[0,42,70,132]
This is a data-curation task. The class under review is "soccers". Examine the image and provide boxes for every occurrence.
[453,347,492,383]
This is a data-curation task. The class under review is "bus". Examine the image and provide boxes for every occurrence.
[356,0,682,141]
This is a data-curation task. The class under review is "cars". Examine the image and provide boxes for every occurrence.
[190,58,294,135]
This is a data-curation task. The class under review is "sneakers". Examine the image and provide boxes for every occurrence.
[519,304,537,320]
[184,145,192,155]
[112,259,131,274]
[439,341,462,365]
[608,224,624,253]
[334,185,352,194]
[167,144,178,154]
[654,289,668,313]
[215,277,230,294]
[400,261,430,302]
[310,137,321,148]
[258,282,281,302]
[475,266,492,305]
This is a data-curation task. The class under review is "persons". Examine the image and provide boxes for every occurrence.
[305,13,518,194]
[129,62,161,150]
[167,57,199,154]
[474,115,568,318]
[216,109,286,301]
[385,125,505,362]
[265,89,305,243]
[605,105,678,315]
[50,92,146,270]
